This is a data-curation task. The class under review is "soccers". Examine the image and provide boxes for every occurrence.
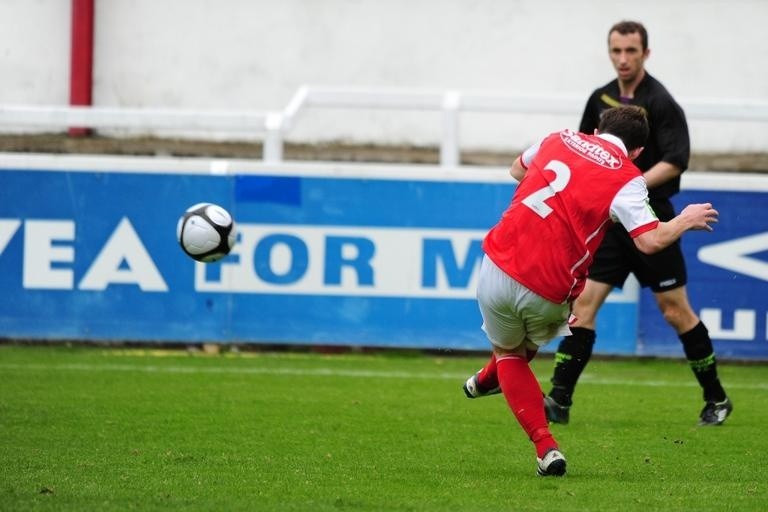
[177,203,236,262]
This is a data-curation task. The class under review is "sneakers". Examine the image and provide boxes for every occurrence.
[542,395,568,423]
[463,373,502,398]
[537,448,566,475]
[698,399,732,426]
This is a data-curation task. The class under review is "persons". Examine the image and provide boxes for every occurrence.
[460,103,724,480]
[542,18,735,430]
